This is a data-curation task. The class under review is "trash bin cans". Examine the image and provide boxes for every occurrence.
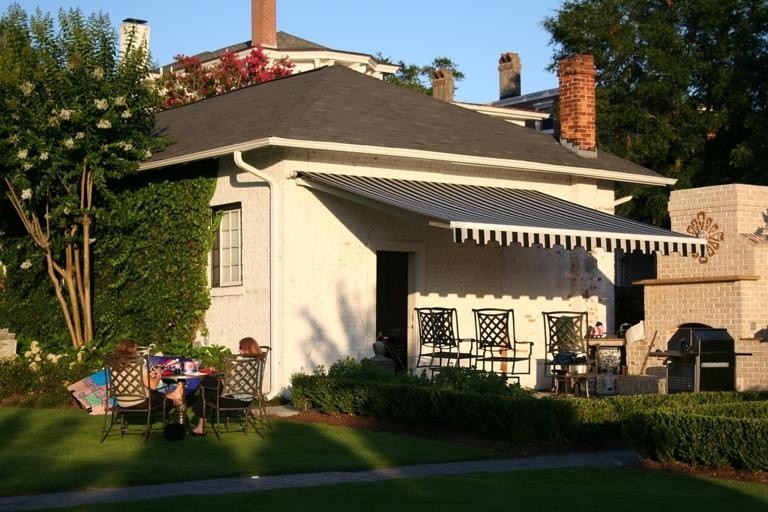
[668,323,736,394]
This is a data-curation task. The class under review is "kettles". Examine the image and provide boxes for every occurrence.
[591,321,603,338]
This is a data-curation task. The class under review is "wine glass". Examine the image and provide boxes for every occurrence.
[184,359,202,374]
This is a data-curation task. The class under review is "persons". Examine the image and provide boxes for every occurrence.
[192,337,265,435]
[110,339,183,409]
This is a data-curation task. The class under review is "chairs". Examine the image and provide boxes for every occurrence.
[541,311,594,400]
[472,306,535,388]
[414,306,482,383]
[199,353,269,438]
[100,353,165,444]
[249,344,275,427]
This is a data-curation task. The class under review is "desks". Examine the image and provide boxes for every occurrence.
[161,373,207,437]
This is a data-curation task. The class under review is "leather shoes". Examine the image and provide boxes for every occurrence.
[189,429,207,436]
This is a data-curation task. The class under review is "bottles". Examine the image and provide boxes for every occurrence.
[155,358,181,376]
